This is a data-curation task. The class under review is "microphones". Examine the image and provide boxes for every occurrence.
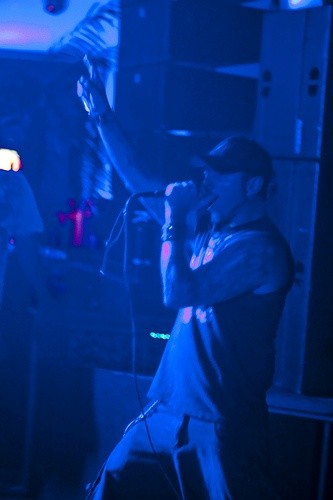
[136,186,208,201]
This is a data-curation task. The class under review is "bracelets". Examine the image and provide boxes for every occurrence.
[89,108,115,124]
[160,224,181,241]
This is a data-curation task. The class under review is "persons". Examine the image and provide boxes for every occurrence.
[0,168,52,303]
[76,53,294,500]
